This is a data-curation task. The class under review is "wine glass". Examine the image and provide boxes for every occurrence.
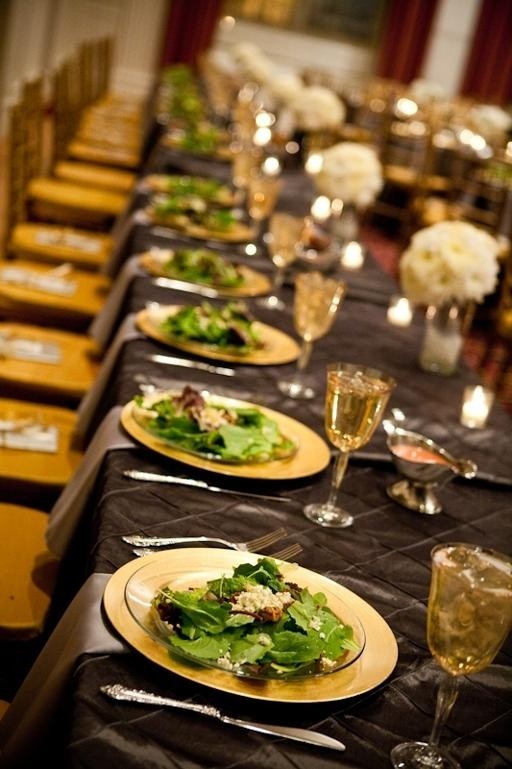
[299,357,400,527]
[395,541,510,769]
[258,211,310,309]
[276,271,346,400]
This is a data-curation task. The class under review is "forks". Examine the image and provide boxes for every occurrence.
[121,527,289,554]
[123,543,303,561]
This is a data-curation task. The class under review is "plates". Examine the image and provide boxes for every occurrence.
[122,389,331,481]
[126,557,367,672]
[104,544,400,701]
[131,57,302,376]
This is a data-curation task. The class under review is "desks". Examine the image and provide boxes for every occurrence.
[1,61,512,769]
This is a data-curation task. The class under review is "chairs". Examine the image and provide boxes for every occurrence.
[0,32,141,720]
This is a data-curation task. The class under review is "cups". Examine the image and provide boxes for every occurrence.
[459,385,495,433]
[385,294,418,326]
[341,240,369,269]
[385,427,477,516]
[337,70,512,244]
[414,290,477,376]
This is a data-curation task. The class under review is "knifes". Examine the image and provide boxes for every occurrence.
[125,470,300,512]
[324,445,511,487]
[102,683,347,754]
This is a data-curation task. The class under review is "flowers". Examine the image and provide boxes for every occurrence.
[282,86,347,132]
[390,209,501,311]
[309,135,391,207]
